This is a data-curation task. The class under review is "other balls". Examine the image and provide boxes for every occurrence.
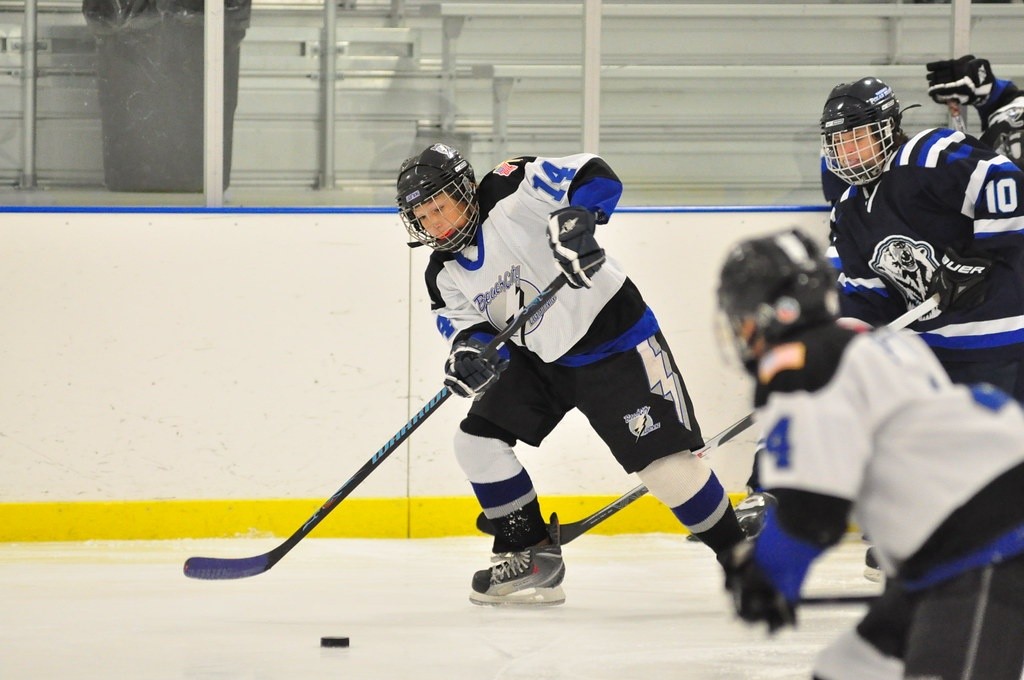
[320,636,350,647]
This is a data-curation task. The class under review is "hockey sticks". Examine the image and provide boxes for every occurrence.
[473,285,967,547]
[182,269,570,580]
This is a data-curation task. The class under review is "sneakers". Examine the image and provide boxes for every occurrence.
[467,511,566,604]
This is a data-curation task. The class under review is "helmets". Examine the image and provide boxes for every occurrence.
[821,76,902,136]
[397,143,478,254]
[717,230,839,343]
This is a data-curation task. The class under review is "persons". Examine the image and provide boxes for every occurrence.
[818,53,1023,580]
[395,144,755,597]
[714,227,1023,679]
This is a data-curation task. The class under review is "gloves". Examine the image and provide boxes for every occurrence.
[926,246,993,317]
[546,205,606,290]
[927,55,994,108]
[444,340,511,398]
[735,560,795,632]
